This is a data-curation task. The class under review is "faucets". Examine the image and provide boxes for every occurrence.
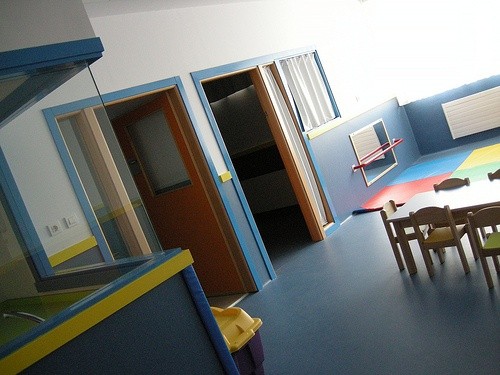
[3,310,45,324]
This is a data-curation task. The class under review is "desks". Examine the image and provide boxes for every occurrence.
[386,177,500,274]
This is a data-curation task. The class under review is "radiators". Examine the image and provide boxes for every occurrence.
[352,126,385,166]
[440,86,500,140]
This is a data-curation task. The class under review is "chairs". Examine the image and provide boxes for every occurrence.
[380,167,500,289]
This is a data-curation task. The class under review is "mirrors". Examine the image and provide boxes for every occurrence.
[348,117,399,187]
